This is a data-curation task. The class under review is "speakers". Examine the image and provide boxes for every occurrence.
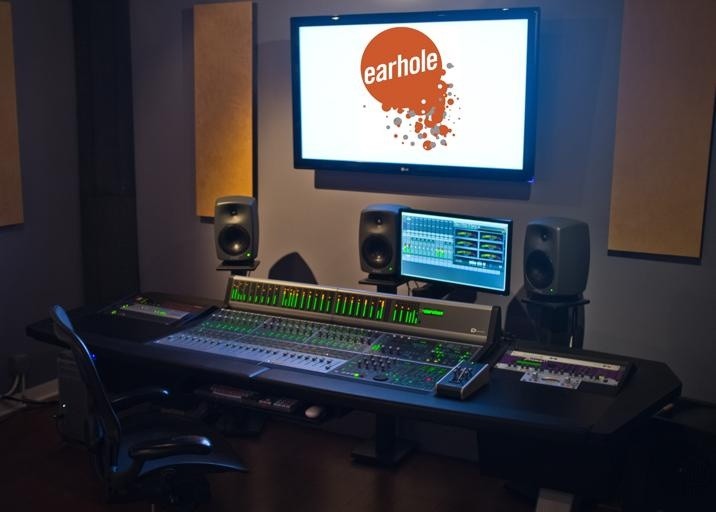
[358,204,411,275]
[524,216,590,297]
[214,196,259,262]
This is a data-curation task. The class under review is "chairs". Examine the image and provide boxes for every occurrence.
[50,304,248,511]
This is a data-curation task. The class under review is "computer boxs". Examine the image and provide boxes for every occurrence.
[57,349,95,449]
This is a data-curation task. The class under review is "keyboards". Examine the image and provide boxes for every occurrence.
[211,384,303,414]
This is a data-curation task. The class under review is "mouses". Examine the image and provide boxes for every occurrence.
[305,405,326,418]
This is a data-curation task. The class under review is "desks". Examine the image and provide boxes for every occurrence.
[27,292,683,512]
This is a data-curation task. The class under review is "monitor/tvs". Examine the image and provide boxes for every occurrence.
[397,209,513,295]
[290,7,540,182]
